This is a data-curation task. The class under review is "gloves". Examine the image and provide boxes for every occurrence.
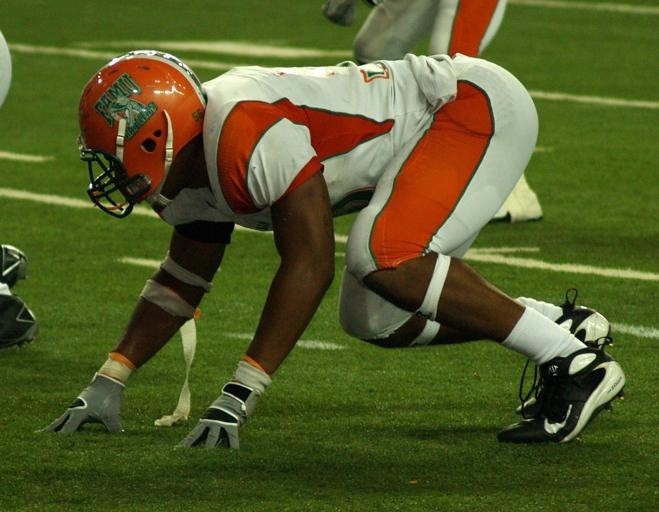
[174,354,282,451]
[34,351,136,436]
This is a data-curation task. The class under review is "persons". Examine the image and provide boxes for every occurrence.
[1,27,42,357]
[31,46,628,450]
[318,0,545,229]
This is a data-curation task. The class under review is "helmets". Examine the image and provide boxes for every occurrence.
[79,49,207,201]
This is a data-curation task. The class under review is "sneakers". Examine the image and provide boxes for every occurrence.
[496,302,626,443]
[488,174,543,224]
[0,244,39,350]
